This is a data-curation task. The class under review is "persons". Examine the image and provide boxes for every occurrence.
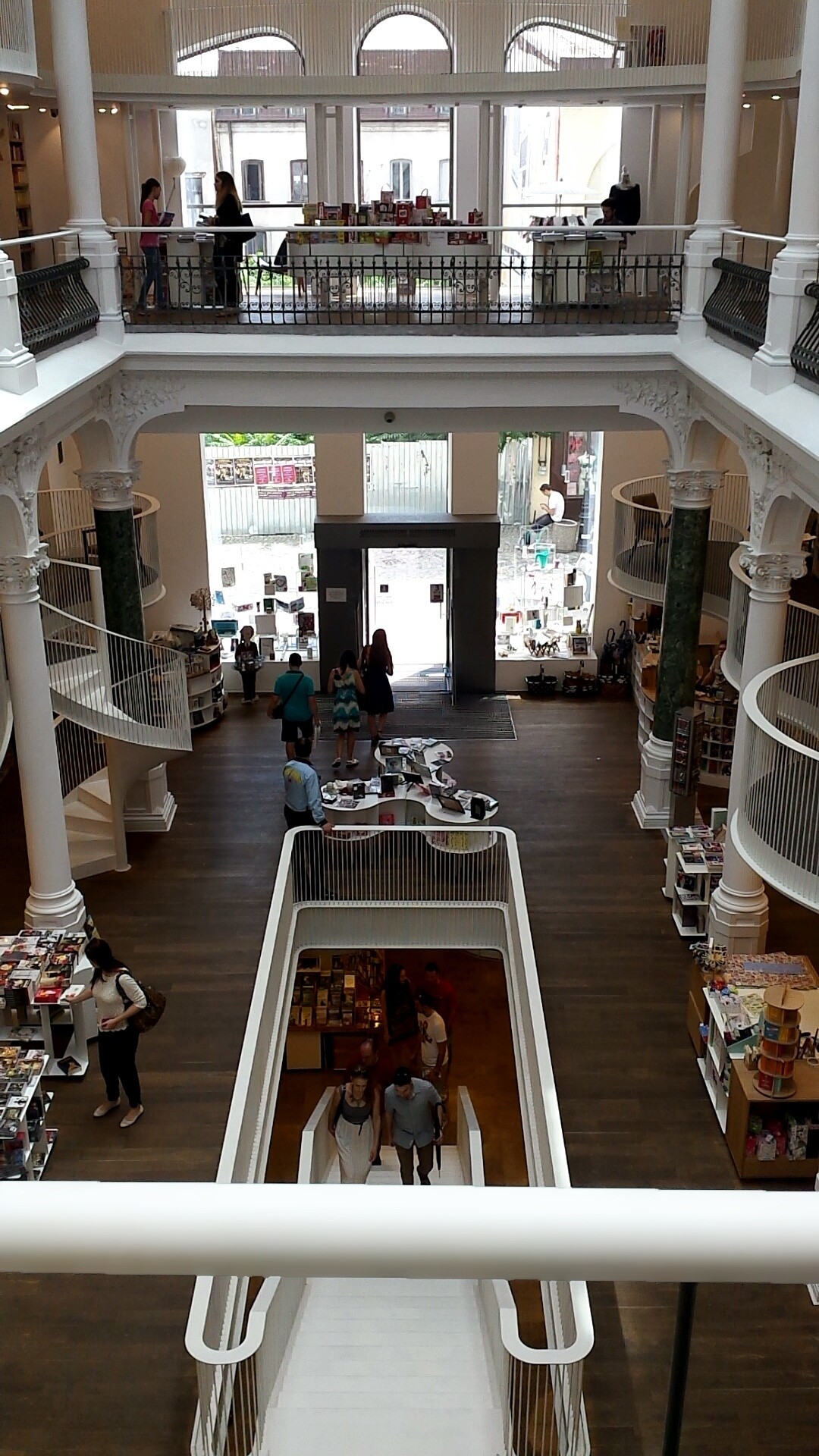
[269,653,320,765]
[414,990,452,1100]
[524,485,565,543]
[384,1070,444,1186]
[383,963,414,1033]
[609,164,641,235]
[350,1038,388,1166]
[418,962,461,1041]
[234,625,260,704]
[281,737,335,834]
[358,629,395,748]
[66,938,148,1126]
[699,641,727,693]
[328,1063,381,1184]
[135,176,170,316]
[327,650,366,768]
[204,171,244,318]
[592,197,628,297]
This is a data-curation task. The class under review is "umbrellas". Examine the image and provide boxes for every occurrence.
[599,619,634,677]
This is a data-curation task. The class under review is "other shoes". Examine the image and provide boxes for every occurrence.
[440,1093,447,1101]
[377,731,383,740]
[371,736,377,745]
[93,1097,120,1117]
[421,1176,431,1185]
[120,1104,144,1127]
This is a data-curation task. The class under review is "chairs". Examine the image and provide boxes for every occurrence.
[83,507,149,578]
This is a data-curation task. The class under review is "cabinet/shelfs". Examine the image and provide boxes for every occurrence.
[143,625,228,734]
[688,955,819,1177]
[320,738,497,867]
[662,826,725,936]
[0,1047,58,1181]
[0,930,99,1075]
[694,700,737,776]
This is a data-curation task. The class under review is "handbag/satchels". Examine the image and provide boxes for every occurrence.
[334,1082,346,1125]
[271,702,285,719]
[360,645,370,677]
[115,971,166,1032]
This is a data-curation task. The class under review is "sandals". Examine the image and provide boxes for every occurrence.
[332,758,341,768]
[346,759,359,768]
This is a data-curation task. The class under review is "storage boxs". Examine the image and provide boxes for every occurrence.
[525,662,629,699]
